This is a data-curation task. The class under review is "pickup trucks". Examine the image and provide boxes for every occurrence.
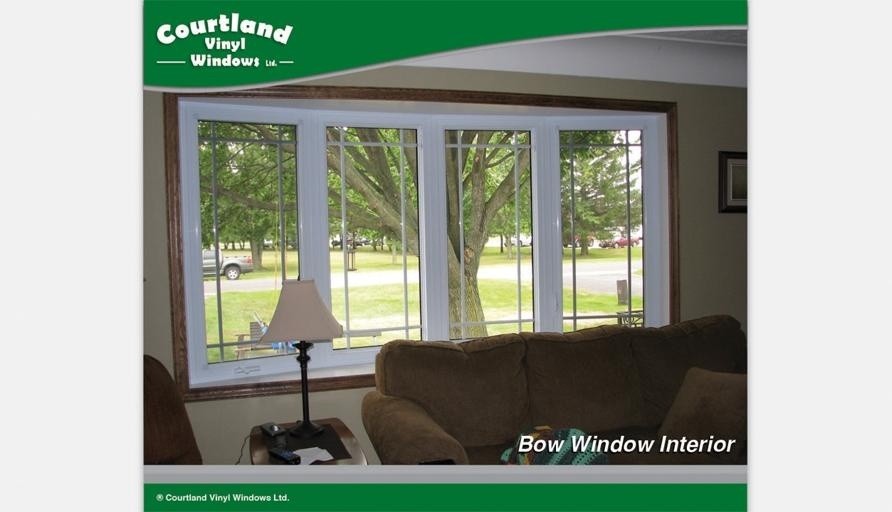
[203,250,253,280]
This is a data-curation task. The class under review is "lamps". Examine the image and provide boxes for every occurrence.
[258,279,344,438]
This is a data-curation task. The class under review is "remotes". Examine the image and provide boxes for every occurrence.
[268,447,301,465]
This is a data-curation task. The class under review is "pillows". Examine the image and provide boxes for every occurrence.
[654,366,745,462]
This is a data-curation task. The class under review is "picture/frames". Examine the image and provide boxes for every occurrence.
[719,150,747,213]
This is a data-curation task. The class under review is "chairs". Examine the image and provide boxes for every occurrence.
[234,322,273,360]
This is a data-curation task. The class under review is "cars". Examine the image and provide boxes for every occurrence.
[263,234,384,248]
[503,230,641,249]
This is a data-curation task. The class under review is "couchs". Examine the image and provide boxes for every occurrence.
[362,315,747,464]
